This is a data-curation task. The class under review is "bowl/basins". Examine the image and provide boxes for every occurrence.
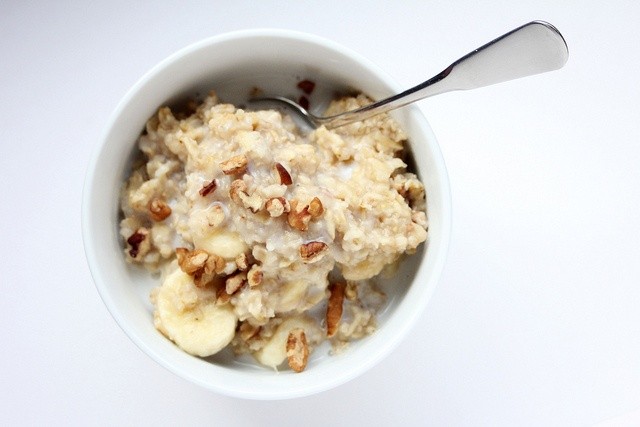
[79,27,453,402]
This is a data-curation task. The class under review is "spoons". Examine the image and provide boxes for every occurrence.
[245,19,569,136]
